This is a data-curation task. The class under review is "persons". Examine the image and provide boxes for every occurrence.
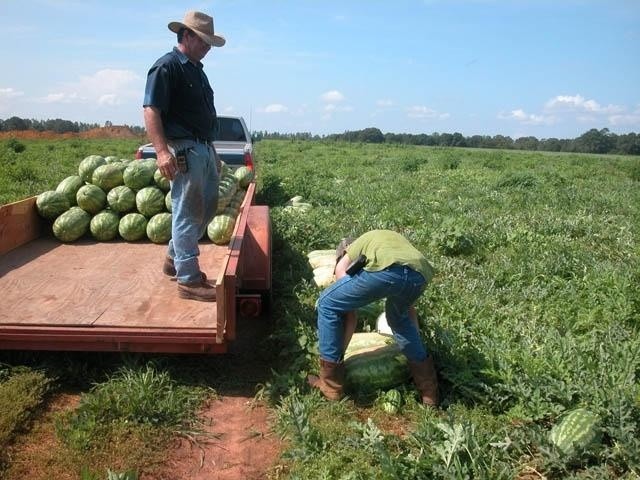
[308,229,440,406]
[143,11,226,302]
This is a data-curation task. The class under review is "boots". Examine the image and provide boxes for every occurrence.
[307,355,347,402]
[408,351,444,408]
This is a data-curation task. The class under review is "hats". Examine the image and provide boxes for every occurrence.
[168,11,226,47]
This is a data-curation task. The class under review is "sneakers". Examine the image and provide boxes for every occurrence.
[175,281,217,302]
[162,255,206,285]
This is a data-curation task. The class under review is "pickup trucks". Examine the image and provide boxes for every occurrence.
[135,111,256,172]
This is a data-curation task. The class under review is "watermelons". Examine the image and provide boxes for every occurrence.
[284,194,313,212]
[35,154,255,245]
[548,407,605,455]
[343,331,408,386]
[307,249,339,286]
[385,387,401,412]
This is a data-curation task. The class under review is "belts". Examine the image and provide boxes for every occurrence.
[184,134,212,146]
